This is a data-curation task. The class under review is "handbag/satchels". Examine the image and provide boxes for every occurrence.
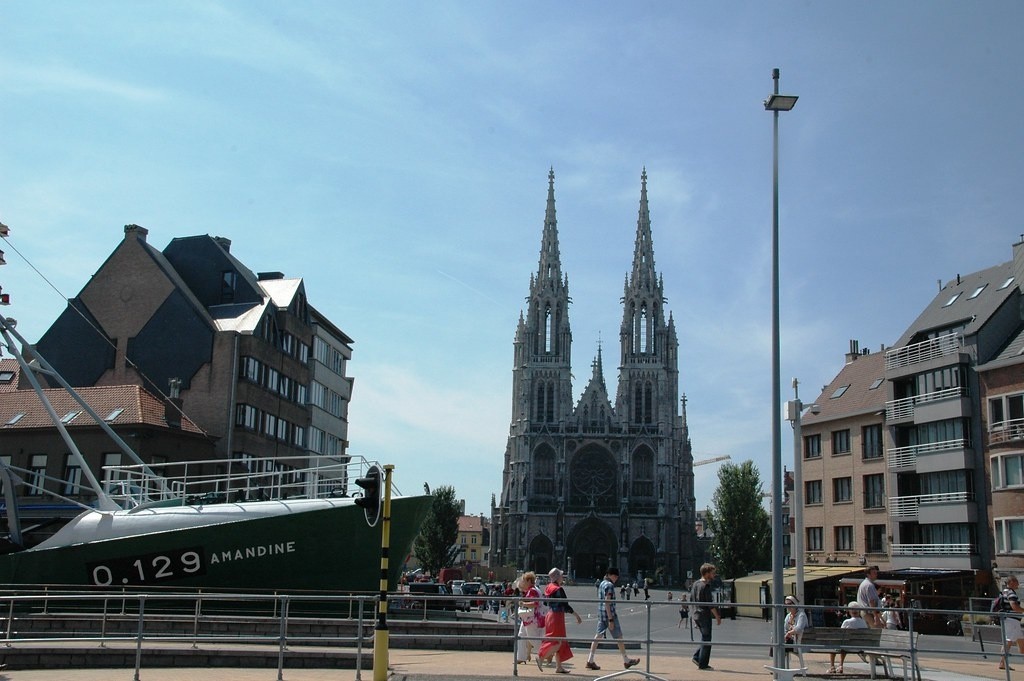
[518,606,534,618]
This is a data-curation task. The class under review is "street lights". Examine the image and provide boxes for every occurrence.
[763,64,798,681]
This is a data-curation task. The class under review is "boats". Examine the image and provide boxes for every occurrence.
[0,223,438,620]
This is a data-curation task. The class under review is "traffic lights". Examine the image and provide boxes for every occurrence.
[353,465,381,527]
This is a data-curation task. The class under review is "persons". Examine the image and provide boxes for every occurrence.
[874,584,920,630]
[595,579,601,588]
[826,602,867,674]
[999,576,1024,671]
[585,567,640,670]
[513,568,581,673]
[477,579,520,614]
[632,581,638,597]
[678,595,689,629]
[769,594,808,658]
[644,585,650,600]
[857,566,883,666]
[620,584,632,600]
[692,563,721,669]
[823,601,851,627]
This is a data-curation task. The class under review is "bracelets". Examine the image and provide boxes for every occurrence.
[609,619,613,622]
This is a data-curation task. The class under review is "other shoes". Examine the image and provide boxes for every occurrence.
[837,666,843,673]
[545,657,552,666]
[858,654,869,664]
[556,667,570,673]
[826,666,836,673]
[698,666,714,670]
[875,659,883,665]
[999,664,1015,670]
[535,656,543,672]
[692,658,699,666]
[585,662,600,670]
[624,658,640,669]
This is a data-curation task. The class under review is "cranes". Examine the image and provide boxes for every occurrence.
[695,454,733,467]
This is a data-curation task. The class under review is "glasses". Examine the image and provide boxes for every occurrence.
[785,602,793,606]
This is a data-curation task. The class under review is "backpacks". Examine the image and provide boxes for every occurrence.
[990,592,1012,626]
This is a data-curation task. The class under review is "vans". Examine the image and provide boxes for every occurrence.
[400,567,505,613]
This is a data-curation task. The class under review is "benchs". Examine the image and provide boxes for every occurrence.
[773,629,886,677]
[866,630,920,681]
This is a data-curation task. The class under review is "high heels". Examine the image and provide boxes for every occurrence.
[513,659,526,664]
[526,657,531,662]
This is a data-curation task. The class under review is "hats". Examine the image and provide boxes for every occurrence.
[606,567,619,576]
[549,567,564,582]
